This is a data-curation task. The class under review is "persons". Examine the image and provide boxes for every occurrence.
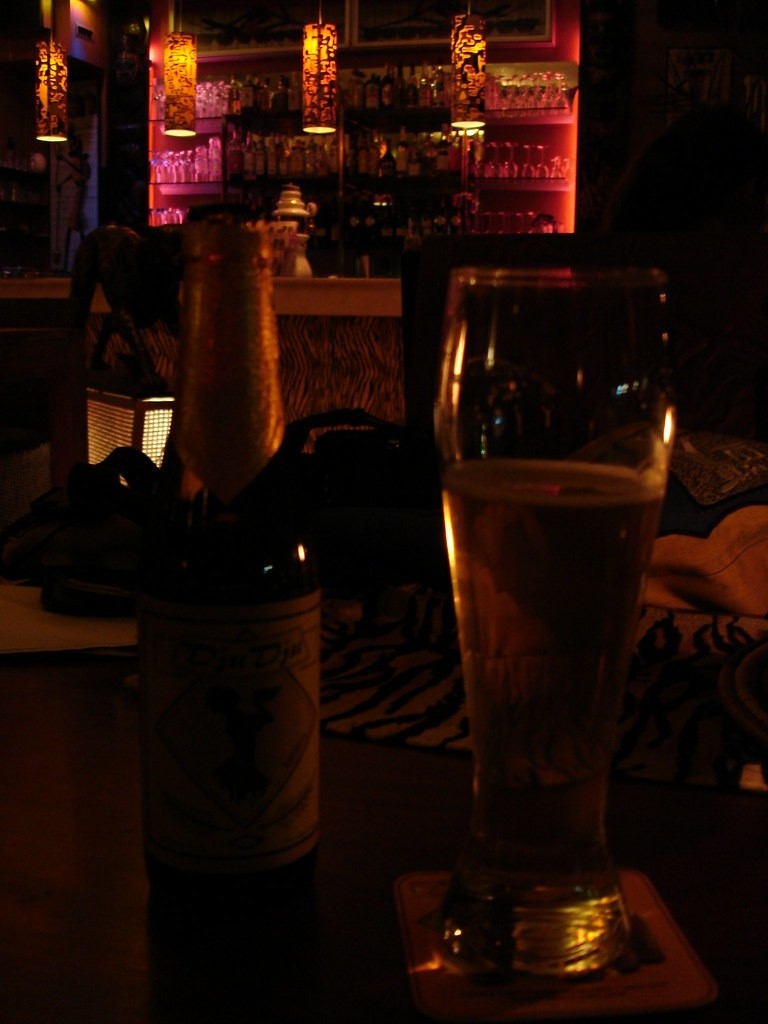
[55,137,92,271]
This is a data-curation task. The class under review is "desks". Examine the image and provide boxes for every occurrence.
[0,665,768,1024]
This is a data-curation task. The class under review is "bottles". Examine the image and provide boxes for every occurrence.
[139,224,321,888]
[241,192,462,243]
[352,60,452,111]
[349,122,456,177]
[226,128,337,179]
[229,74,302,115]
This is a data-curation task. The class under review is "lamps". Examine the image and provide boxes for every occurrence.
[35,0,71,142]
[162,0,197,138]
[301,0,339,136]
[448,0,487,128]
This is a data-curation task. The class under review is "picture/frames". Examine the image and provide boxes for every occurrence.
[350,0,557,50]
[173,0,350,64]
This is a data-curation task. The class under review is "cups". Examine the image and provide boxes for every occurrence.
[149,208,189,226]
[434,269,671,973]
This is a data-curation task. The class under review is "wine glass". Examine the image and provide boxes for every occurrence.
[472,140,570,178]
[478,209,555,235]
[492,73,569,111]
[153,137,222,184]
[156,81,227,117]
